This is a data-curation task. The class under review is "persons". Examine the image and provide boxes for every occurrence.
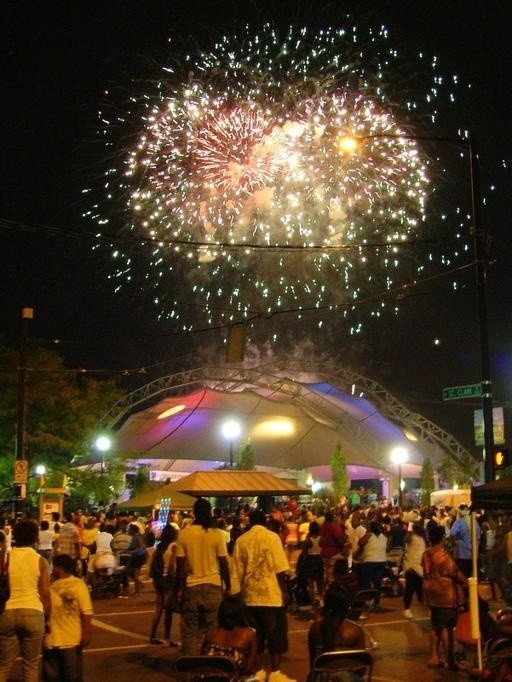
[0,485,512,682]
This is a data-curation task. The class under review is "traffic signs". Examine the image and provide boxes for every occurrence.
[441,382,482,403]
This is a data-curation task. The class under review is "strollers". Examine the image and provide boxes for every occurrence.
[450,581,509,682]
[377,561,404,596]
[84,553,130,601]
[286,573,321,613]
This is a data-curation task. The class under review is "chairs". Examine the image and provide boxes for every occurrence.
[312,649,373,682]
[176,655,243,681]
[347,588,379,627]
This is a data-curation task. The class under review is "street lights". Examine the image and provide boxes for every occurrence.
[333,129,499,490]
[97,435,111,512]
[390,444,406,507]
[222,419,241,510]
